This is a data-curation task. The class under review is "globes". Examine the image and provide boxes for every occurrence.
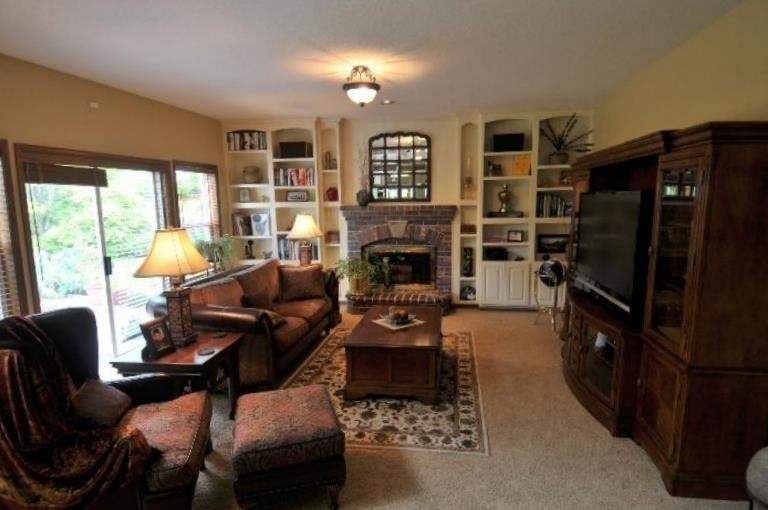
[538,260,564,286]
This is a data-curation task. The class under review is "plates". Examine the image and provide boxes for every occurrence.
[378,314,418,326]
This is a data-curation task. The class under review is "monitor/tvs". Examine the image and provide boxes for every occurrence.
[565,187,648,320]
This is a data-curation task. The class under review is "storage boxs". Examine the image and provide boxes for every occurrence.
[279,141,313,157]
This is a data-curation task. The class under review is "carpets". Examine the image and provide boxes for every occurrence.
[277,325,489,457]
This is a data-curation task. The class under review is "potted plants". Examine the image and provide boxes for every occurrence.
[539,113,596,165]
[194,235,243,272]
[332,258,390,296]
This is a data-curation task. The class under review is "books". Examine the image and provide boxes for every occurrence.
[537,193,565,217]
[274,165,314,186]
[278,238,300,260]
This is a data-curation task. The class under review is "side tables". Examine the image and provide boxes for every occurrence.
[110,329,245,452]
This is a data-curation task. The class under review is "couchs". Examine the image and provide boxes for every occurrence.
[0,306,213,509]
[146,264,340,387]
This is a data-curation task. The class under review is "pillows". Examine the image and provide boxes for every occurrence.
[278,265,325,301]
[209,303,287,329]
[69,376,133,427]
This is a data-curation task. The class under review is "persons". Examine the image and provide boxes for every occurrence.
[151,328,170,351]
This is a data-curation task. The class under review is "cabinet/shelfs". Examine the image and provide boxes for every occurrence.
[563,292,641,438]
[222,117,341,267]
[635,340,768,499]
[450,114,592,309]
[645,142,768,371]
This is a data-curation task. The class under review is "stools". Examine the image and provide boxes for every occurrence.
[232,384,346,509]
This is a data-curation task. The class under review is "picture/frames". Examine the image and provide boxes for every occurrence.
[139,317,175,359]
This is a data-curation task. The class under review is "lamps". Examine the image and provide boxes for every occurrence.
[285,214,323,264]
[134,230,211,348]
[342,66,380,106]
